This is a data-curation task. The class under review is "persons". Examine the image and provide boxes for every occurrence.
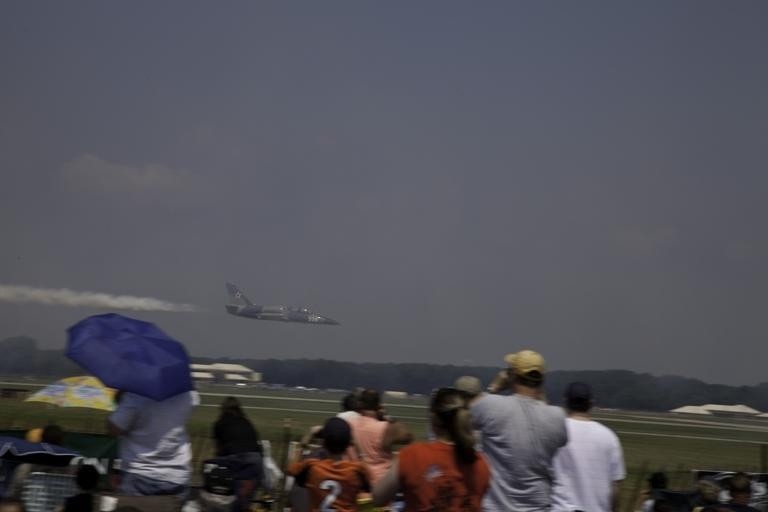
[1,376,491,510]
[633,471,757,511]
[457,349,626,511]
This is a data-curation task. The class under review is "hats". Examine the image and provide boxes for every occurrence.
[505,349,547,383]
[454,374,483,396]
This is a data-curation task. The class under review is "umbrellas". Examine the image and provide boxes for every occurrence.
[60,312,195,401]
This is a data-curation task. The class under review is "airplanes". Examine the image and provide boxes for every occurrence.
[222,282,342,326]
